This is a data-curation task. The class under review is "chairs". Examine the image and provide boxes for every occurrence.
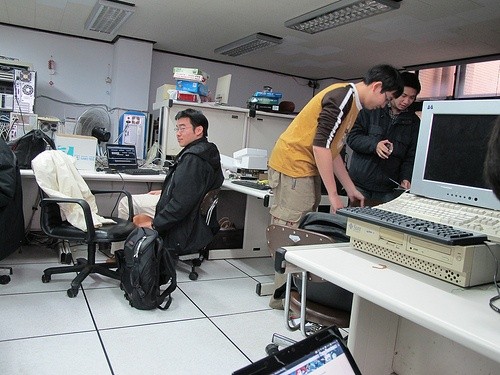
[31,149,138,299]
[174,186,223,282]
[266,223,353,337]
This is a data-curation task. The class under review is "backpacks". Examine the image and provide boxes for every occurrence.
[114,227,177,311]
[6,128,56,170]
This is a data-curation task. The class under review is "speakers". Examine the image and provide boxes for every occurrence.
[37,120,60,143]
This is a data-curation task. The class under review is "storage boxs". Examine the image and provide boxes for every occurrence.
[177,91,212,103]
[173,72,206,85]
[232,147,267,159]
[247,97,279,105]
[175,80,210,97]
[172,66,210,80]
[53,131,98,171]
[239,155,267,167]
[247,102,280,113]
[254,90,282,99]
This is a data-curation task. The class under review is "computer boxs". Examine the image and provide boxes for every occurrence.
[13,69,36,113]
[8,112,38,143]
[346,218,500,287]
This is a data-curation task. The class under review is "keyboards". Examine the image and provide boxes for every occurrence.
[124,168,159,175]
[335,205,488,246]
[231,180,271,190]
[370,192,500,243]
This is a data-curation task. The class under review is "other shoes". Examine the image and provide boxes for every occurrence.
[269,296,291,311]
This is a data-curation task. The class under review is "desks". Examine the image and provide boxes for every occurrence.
[64,172,199,264]
[271,243,500,375]
[204,176,349,261]
[19,168,65,264]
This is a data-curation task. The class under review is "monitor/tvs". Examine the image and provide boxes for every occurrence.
[409,98,500,212]
[229,324,364,375]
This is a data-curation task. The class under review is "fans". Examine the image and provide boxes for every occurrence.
[73,106,112,146]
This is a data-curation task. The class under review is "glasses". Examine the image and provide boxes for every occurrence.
[384,92,391,107]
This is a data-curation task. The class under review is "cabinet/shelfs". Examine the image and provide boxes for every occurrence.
[153,99,297,165]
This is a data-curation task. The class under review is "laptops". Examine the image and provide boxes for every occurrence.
[105,143,138,173]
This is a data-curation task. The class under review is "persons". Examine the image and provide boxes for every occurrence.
[268,64,404,311]
[106,108,223,264]
[345,71,422,207]
[0,136,17,212]
